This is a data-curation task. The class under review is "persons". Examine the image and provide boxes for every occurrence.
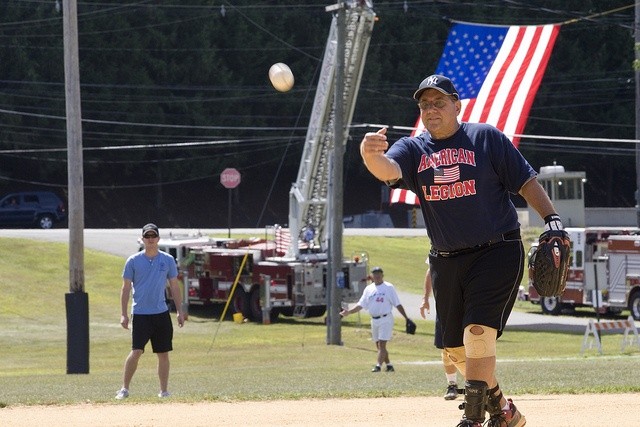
[115,224,185,399]
[339,267,416,373]
[6,198,17,206]
[420,257,458,400]
[360,75,571,427]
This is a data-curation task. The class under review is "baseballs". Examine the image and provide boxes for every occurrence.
[269,62,295,91]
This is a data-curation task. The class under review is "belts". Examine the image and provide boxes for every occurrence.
[430,230,520,258]
[372,314,387,319]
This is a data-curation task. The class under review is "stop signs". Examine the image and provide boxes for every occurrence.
[220,167,242,190]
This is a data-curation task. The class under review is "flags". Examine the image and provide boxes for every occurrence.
[382,21,561,205]
[277,228,313,252]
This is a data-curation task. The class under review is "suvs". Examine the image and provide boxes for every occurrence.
[0,191,67,229]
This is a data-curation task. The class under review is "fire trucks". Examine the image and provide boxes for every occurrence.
[518,224,640,322]
[138,0,379,321]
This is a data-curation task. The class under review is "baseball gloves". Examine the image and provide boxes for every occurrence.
[406,320,416,335]
[528,230,570,297]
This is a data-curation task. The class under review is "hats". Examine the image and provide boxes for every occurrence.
[142,224,159,237]
[371,267,383,273]
[413,75,459,100]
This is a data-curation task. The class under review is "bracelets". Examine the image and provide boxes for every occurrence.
[543,213,561,223]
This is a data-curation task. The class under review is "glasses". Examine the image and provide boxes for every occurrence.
[144,234,157,238]
[418,99,447,110]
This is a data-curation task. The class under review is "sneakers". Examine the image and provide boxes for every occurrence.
[372,366,381,372]
[115,388,129,400]
[456,420,482,427]
[385,365,394,372]
[158,392,171,397]
[483,398,526,427]
[444,384,457,400]
